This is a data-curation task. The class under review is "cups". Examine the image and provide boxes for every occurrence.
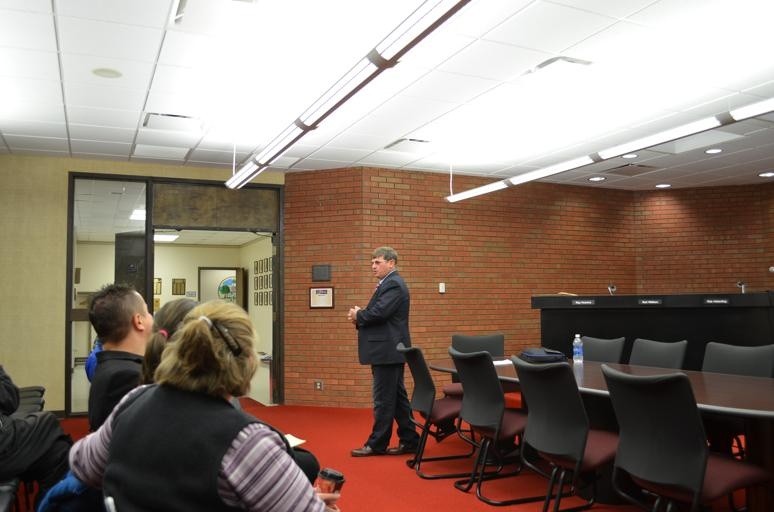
[314,467,344,507]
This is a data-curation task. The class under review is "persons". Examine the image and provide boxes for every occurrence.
[347,246,425,458]
[66,281,340,512]
[0,363,73,510]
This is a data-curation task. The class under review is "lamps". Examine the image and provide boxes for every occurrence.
[224,0,470,192]
[444,93,774,204]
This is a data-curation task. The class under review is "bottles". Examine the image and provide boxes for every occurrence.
[572,335,583,363]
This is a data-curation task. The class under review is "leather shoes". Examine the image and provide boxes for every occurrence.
[352,445,386,457]
[388,443,416,455]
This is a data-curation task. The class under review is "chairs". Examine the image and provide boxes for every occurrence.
[451,334,505,357]
[395,341,508,479]
[0,381,47,512]
[701,340,773,381]
[509,352,623,511]
[600,361,765,512]
[629,336,689,370]
[446,345,580,506]
[580,335,626,365]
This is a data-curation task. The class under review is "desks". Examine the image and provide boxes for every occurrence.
[427,357,774,427]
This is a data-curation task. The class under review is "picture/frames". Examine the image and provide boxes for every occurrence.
[253,257,273,306]
[311,289,336,309]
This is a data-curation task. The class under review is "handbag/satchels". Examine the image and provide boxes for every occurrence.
[519,347,566,363]
